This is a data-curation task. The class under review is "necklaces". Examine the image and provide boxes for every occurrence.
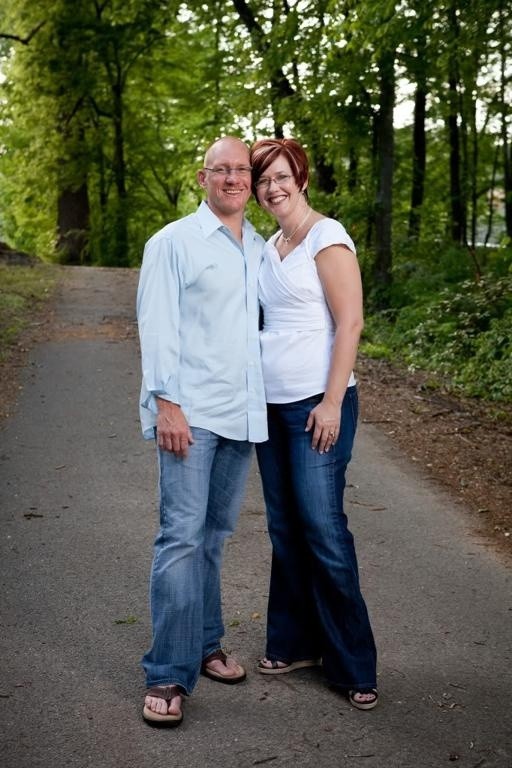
[280,207,313,244]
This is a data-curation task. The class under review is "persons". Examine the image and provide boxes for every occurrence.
[135,135,269,725]
[249,139,380,712]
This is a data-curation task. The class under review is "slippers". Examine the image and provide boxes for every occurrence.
[200,648,246,684]
[142,684,183,728]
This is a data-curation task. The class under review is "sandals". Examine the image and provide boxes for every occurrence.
[348,688,380,710]
[257,654,322,675]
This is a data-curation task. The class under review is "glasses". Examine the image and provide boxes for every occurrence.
[254,173,294,190]
[203,166,253,175]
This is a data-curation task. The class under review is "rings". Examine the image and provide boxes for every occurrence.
[330,430,336,438]
